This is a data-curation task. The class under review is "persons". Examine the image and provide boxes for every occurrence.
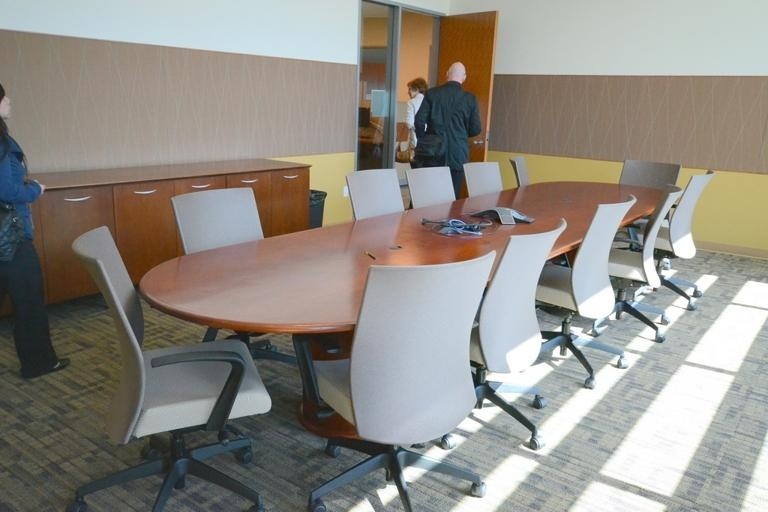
[0,86,70,380]
[409,61,481,210]
[406,78,430,159]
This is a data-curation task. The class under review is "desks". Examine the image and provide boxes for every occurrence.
[140,181,667,443]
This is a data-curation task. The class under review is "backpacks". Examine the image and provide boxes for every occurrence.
[0,200,26,265]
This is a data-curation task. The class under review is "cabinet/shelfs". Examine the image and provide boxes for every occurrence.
[226,171,271,239]
[113,180,177,287]
[174,175,226,258]
[270,167,310,236]
[0,199,48,318]
[39,184,114,305]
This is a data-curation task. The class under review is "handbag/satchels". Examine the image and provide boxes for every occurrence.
[394,140,416,164]
[414,133,447,162]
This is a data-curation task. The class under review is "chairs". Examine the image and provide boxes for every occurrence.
[298,250,496,512]
[614,169,717,312]
[67,225,272,511]
[437,218,568,451]
[463,161,503,197]
[618,159,682,271]
[509,156,530,188]
[170,187,298,365]
[592,183,684,344]
[535,194,638,389]
[404,166,456,209]
[345,168,405,221]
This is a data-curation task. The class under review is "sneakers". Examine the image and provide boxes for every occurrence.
[42,358,70,374]
